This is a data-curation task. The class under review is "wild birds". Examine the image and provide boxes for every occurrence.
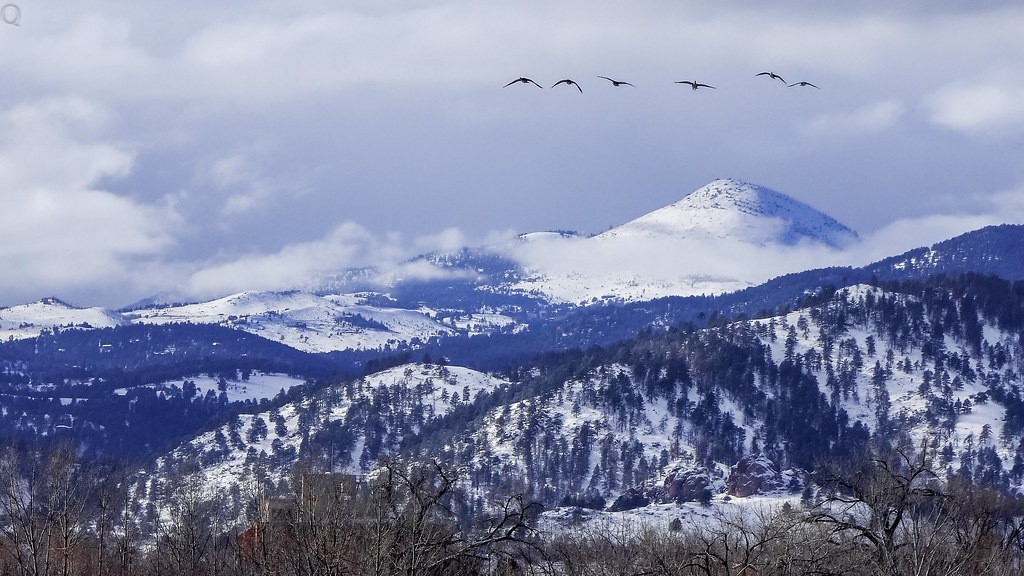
[551,78,584,95]
[596,74,636,89]
[755,71,822,90]
[502,76,544,90]
[673,79,717,92]
